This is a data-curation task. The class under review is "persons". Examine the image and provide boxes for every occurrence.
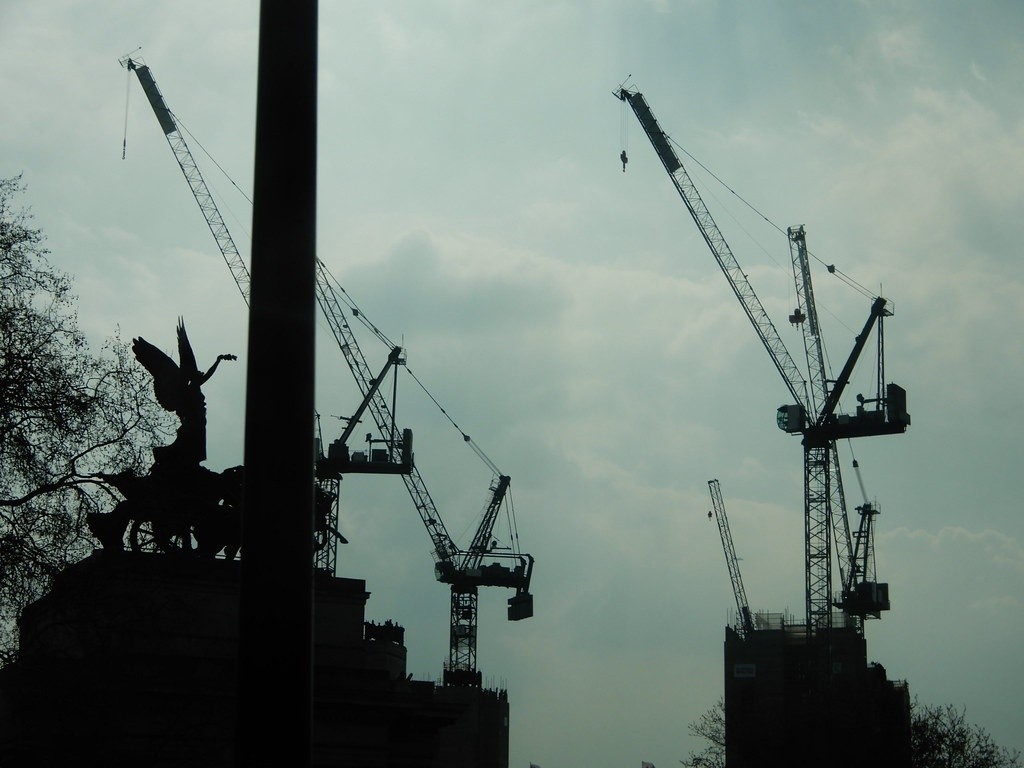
[130,314,239,470]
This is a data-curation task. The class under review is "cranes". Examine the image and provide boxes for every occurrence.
[118,56,534,689]
[614,87,911,768]
